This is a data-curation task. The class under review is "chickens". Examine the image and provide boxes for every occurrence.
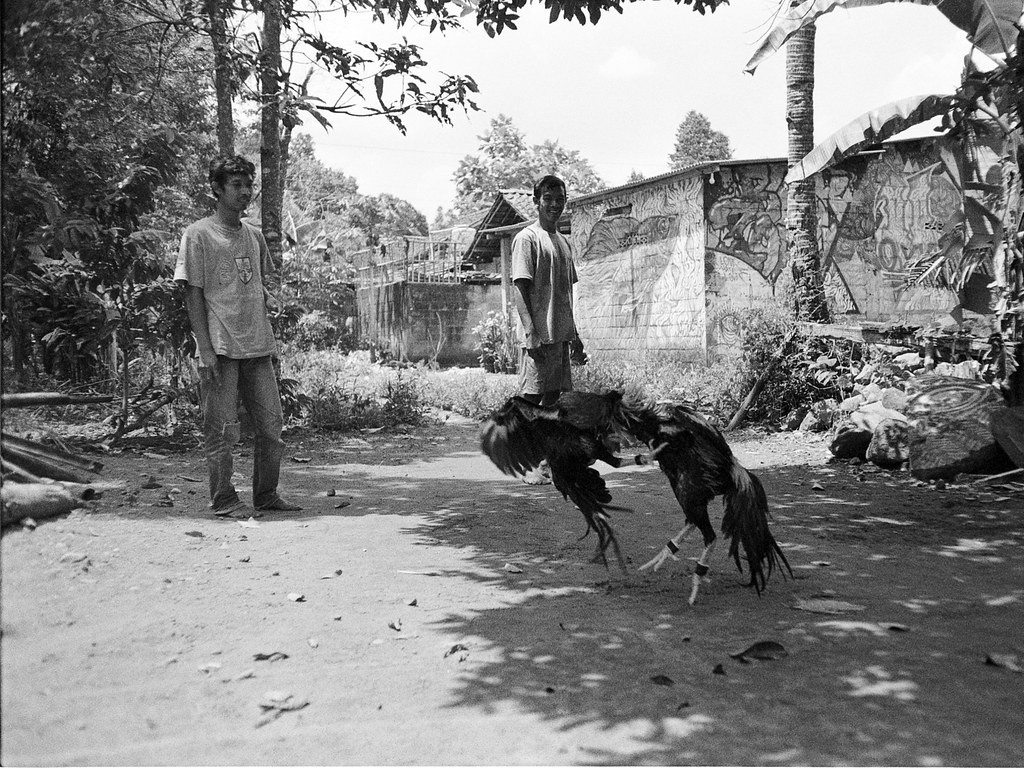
[480,387,796,607]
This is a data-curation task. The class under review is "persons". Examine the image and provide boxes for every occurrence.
[512,175,590,488]
[172,155,304,521]
